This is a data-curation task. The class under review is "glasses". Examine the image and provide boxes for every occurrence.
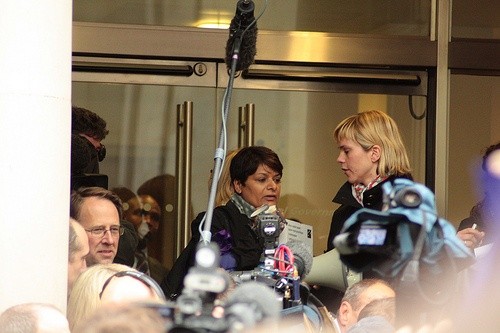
[86,225,125,236]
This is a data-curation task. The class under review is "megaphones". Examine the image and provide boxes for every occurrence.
[301,247,363,294]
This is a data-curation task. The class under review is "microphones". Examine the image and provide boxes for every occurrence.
[225,0,258,72]
